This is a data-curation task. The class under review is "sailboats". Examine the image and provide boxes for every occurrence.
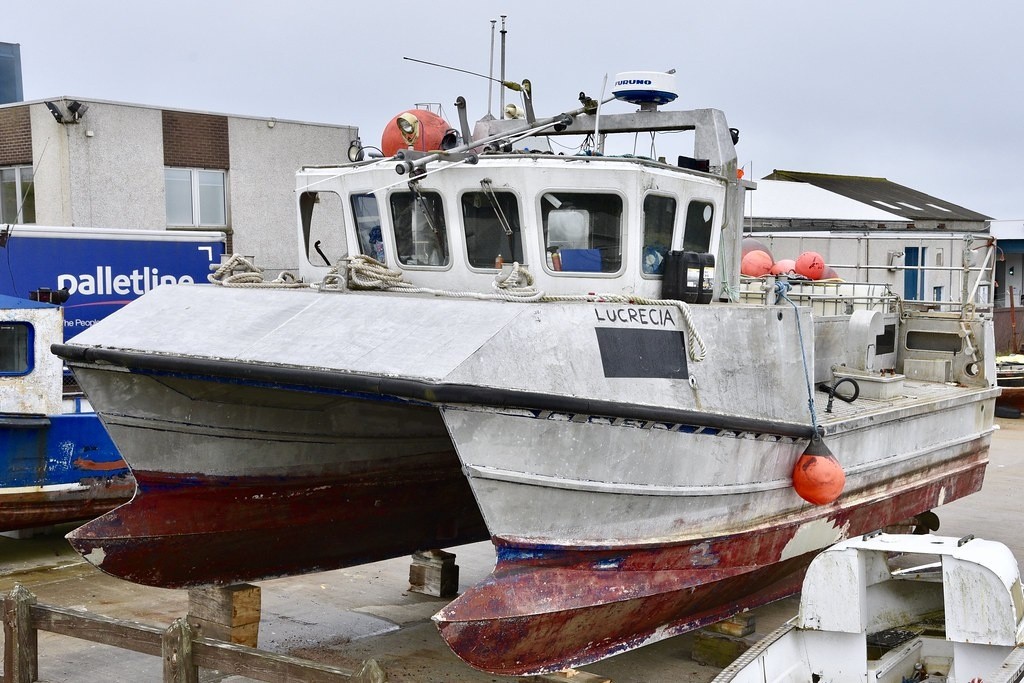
[993,284,1023,413]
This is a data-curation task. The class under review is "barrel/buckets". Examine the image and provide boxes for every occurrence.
[220,254,255,281]
[697,253,715,304]
[675,251,701,304]
[661,250,683,299]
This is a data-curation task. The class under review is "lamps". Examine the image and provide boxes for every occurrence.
[44,100,64,125]
[67,101,89,121]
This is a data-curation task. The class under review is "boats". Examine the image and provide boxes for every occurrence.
[0,287,138,532]
[708,512,1024,683]
[46,15,1004,683]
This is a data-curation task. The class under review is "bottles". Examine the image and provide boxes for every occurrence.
[495,254,503,269]
